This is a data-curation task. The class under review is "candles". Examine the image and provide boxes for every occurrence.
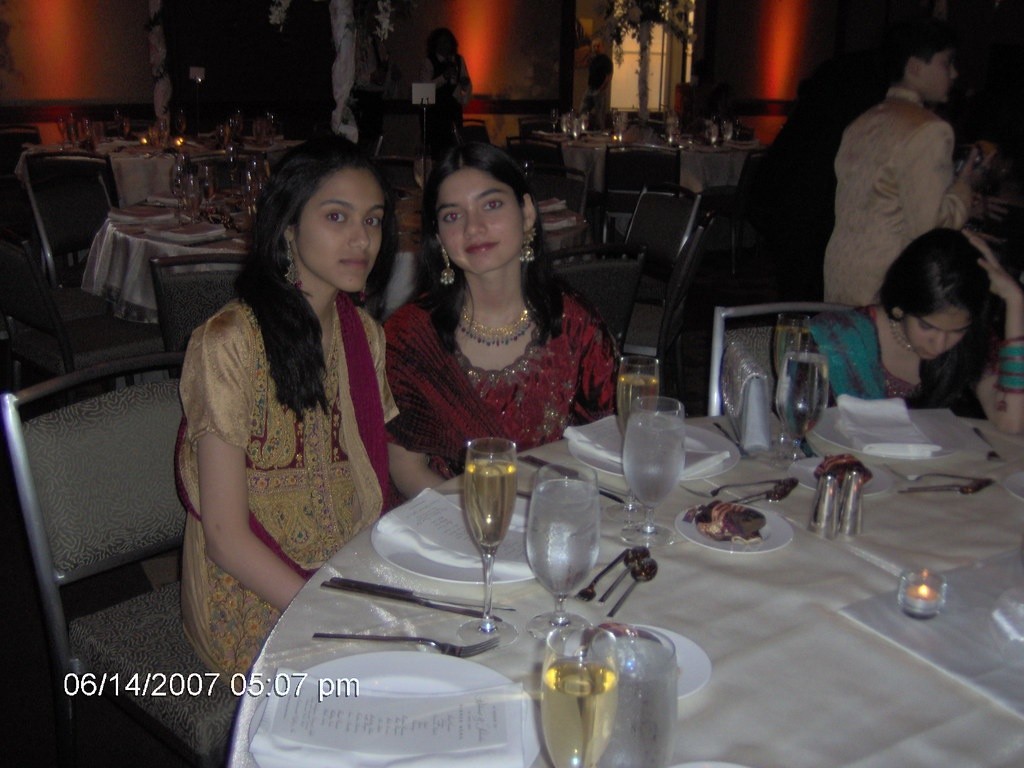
[907,584,939,610]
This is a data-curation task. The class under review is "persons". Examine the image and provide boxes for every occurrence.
[324,66,402,153]
[675,62,722,132]
[418,26,472,155]
[808,230,1023,434]
[382,140,620,497]
[817,27,996,305]
[581,37,611,129]
[173,134,399,689]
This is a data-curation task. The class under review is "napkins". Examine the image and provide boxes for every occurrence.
[377,487,538,578]
[247,666,531,767]
[542,215,576,229]
[536,197,568,212]
[108,206,173,220]
[145,194,182,204]
[161,224,226,240]
[533,129,569,141]
[724,139,759,150]
[694,143,723,153]
[562,415,730,477]
[838,394,943,458]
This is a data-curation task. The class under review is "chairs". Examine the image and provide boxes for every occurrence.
[1,125,1024,741]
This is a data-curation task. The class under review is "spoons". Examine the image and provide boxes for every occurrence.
[598,545,650,603]
[727,476,799,505]
[605,557,657,618]
[898,478,993,494]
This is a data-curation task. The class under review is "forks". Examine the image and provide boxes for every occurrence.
[679,478,779,498]
[882,466,980,481]
[313,632,500,658]
[573,548,630,601]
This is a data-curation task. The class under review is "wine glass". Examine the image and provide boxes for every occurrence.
[527,460,601,642]
[770,315,813,451]
[458,437,520,645]
[57,109,275,228]
[622,395,686,546]
[774,351,828,470]
[607,353,660,522]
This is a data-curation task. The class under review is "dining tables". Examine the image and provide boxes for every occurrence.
[531,132,754,193]
[24,142,305,206]
[225,414,1024,768]
[82,194,586,324]
[461,113,546,146]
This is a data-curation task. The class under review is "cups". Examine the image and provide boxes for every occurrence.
[540,627,618,768]
[552,108,739,148]
[581,636,678,768]
[897,569,946,619]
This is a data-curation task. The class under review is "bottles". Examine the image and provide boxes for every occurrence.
[808,469,841,539]
[838,462,864,537]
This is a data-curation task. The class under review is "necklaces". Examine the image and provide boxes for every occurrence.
[459,302,533,346]
[890,320,913,352]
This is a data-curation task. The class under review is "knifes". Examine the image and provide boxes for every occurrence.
[518,455,624,504]
[322,582,502,622]
[330,577,517,612]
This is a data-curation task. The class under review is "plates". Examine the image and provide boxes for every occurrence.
[249,654,542,768]
[675,503,794,554]
[372,494,537,583]
[561,624,712,705]
[569,424,739,480]
[813,407,964,460]
[788,455,890,497]
[1004,472,1024,499]
